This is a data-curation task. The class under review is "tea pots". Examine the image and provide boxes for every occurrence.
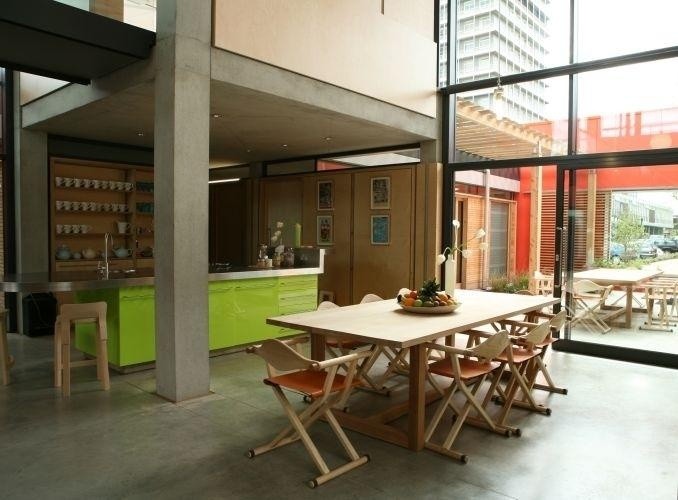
[118,221,131,233]
[56,244,132,260]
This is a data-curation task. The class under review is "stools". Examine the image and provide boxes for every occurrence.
[52,302,109,397]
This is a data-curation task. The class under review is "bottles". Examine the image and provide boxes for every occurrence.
[273,252,281,266]
[287,247,294,266]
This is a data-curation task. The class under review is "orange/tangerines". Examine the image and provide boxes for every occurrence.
[406,297,414,307]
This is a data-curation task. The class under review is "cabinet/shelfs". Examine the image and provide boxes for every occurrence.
[209,274,318,351]
[49,155,154,273]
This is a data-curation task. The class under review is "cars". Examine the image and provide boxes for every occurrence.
[610,242,627,264]
[636,244,658,259]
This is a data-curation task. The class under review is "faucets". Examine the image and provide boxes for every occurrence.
[103,232,114,280]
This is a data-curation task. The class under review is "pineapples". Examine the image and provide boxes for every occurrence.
[419,276,441,302]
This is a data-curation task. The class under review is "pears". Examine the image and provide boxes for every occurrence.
[413,299,423,308]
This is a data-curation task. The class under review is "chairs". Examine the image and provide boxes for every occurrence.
[534,268,678,338]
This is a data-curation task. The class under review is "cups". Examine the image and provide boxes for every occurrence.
[137,181,153,193]
[56,223,95,235]
[257,258,265,268]
[136,223,152,232]
[56,201,128,213]
[53,175,134,193]
[266,259,272,268]
[136,202,154,213]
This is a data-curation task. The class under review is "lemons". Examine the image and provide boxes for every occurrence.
[445,294,447,306]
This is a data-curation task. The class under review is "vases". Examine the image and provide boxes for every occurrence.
[445,254,457,297]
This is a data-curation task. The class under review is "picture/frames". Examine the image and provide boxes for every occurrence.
[316,179,336,247]
[369,176,392,247]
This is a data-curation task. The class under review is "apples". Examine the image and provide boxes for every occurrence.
[408,291,418,300]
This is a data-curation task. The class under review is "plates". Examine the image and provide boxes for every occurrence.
[397,301,457,313]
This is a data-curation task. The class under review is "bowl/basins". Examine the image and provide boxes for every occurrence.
[282,262,288,267]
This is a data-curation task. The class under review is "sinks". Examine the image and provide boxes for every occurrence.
[107,269,136,273]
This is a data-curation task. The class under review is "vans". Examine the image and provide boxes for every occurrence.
[653,239,677,253]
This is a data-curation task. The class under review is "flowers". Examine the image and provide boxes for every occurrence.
[436,218,489,267]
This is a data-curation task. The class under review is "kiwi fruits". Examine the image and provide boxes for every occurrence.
[432,300,439,307]
[423,300,433,308]
[439,300,447,306]
[437,293,448,301]
